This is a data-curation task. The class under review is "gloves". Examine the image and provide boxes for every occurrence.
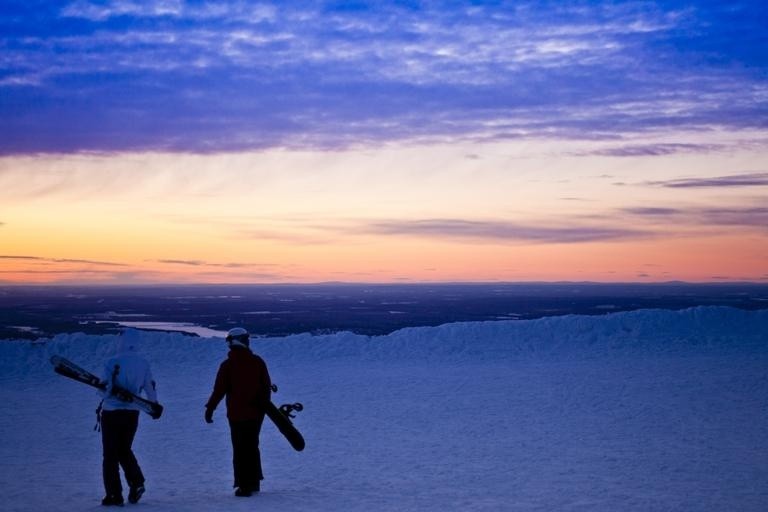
[205,409,214,423]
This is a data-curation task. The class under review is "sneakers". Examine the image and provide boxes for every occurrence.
[128,484,146,505]
[101,494,124,506]
[235,484,252,497]
[252,480,261,491]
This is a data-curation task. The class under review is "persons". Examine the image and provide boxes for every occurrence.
[205,328,271,497]
[96,328,157,505]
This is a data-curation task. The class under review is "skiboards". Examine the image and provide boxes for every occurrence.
[49,352,163,420]
[264,401,305,452]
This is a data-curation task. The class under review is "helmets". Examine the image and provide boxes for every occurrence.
[224,327,250,349]
